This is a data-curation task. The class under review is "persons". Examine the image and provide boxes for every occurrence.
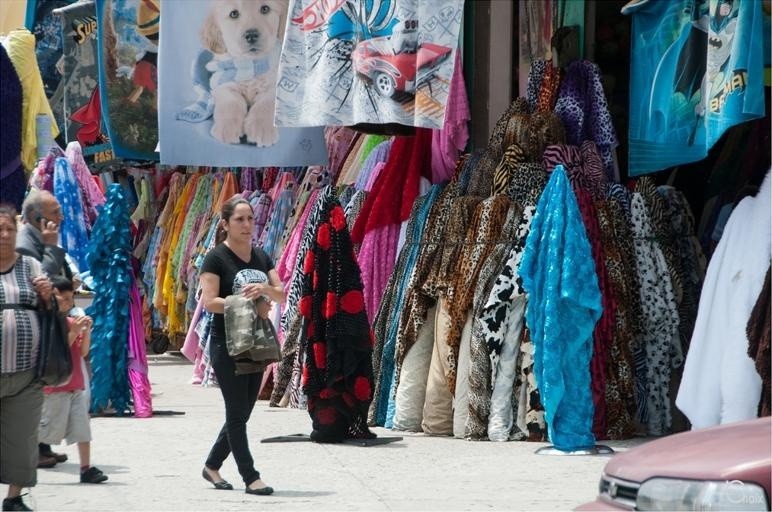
[39,273,109,486]
[708,0,736,33]
[13,188,86,468]
[0,203,69,511]
[198,197,285,496]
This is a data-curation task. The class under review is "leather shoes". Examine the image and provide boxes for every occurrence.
[202,468,233,490]
[246,485,274,495]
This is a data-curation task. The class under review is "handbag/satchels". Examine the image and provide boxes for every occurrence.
[33,295,73,387]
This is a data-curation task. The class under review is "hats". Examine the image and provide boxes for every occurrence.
[136,0,160,37]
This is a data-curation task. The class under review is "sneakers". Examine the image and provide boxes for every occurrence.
[80,467,107,484]
[2,495,33,512]
[37,442,68,468]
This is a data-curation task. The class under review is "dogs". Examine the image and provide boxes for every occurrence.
[200,0,290,147]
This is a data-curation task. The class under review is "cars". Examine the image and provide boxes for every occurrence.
[569,414,772,509]
[351,34,454,99]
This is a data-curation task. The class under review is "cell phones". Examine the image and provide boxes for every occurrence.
[35,215,49,227]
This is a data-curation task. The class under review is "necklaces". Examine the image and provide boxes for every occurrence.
[223,239,253,259]
